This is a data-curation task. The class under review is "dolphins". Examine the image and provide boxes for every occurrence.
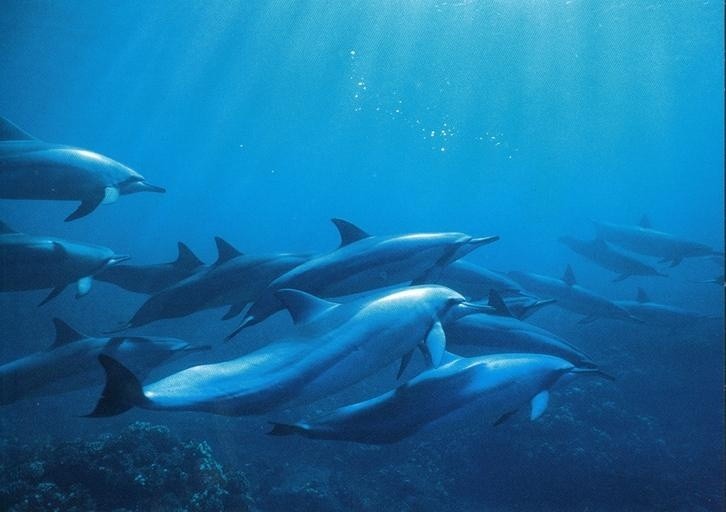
[0,212,725,448]
